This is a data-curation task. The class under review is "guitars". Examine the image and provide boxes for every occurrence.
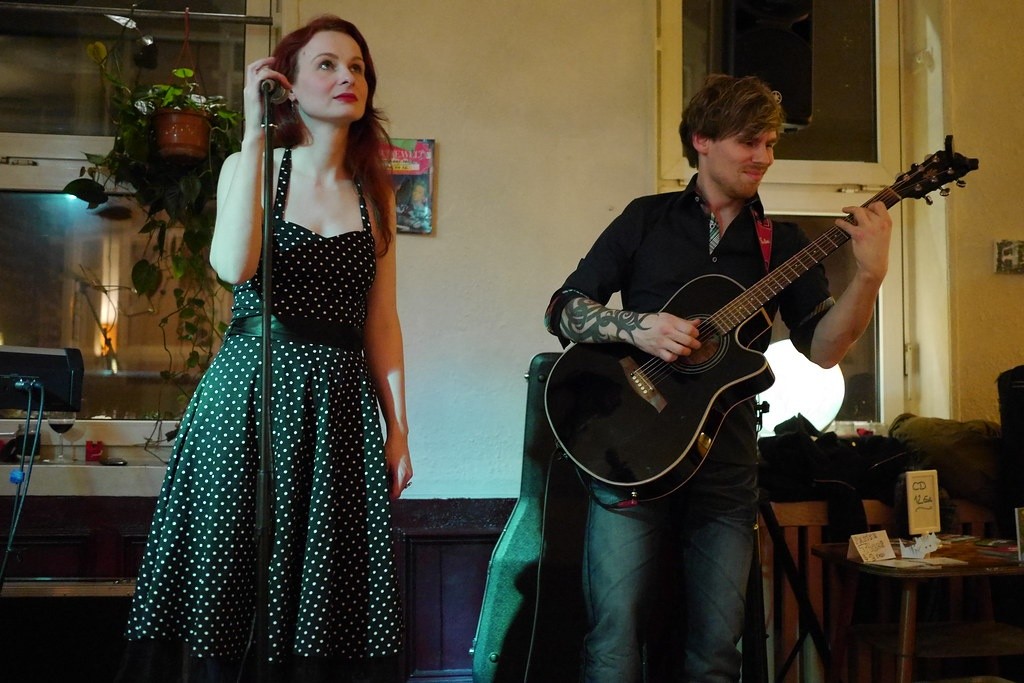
[542,132,981,505]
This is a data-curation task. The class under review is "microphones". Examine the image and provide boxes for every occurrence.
[261,79,289,104]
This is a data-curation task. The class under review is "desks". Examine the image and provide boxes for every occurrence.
[810,544,1024,683]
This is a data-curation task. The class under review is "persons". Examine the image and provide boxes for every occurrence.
[544,76,980,683]
[122,15,413,683]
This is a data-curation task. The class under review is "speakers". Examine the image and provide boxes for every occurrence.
[721,0,814,131]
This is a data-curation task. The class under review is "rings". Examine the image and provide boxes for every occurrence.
[405,482,412,488]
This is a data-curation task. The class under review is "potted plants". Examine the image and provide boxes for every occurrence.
[63,42,244,350]
